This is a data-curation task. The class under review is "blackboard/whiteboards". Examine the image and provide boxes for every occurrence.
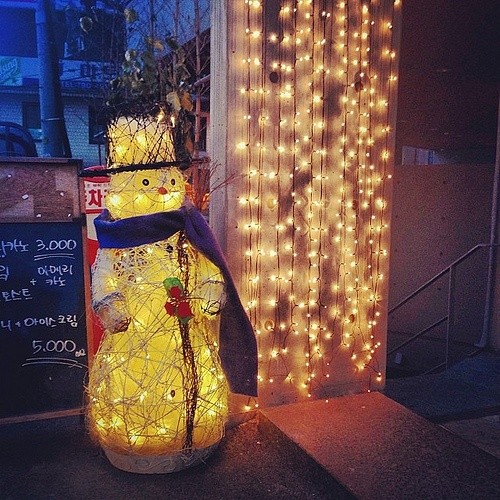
[0,217,95,426]
